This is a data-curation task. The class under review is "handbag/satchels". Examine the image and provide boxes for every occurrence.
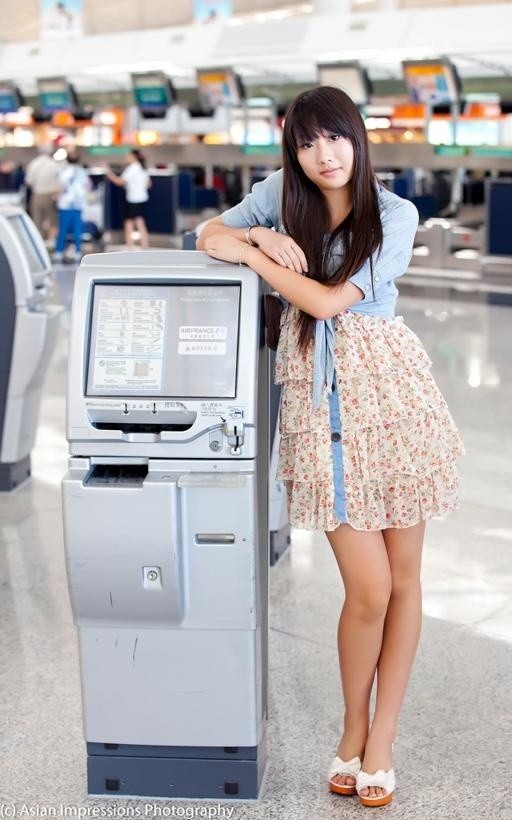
[263,291,286,352]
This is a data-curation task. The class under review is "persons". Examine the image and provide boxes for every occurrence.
[194,85,465,807]
[49,152,93,261]
[24,141,61,253]
[101,150,152,250]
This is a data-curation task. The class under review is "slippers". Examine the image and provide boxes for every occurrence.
[355,766,396,808]
[327,756,362,796]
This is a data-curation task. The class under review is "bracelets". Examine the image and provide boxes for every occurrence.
[239,246,247,267]
[245,224,258,247]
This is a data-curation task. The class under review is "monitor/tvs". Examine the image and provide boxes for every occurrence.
[399,59,458,107]
[0,77,23,115]
[315,60,370,111]
[128,70,174,119]
[3,209,53,282]
[32,76,78,123]
[191,65,239,115]
[78,276,243,404]
